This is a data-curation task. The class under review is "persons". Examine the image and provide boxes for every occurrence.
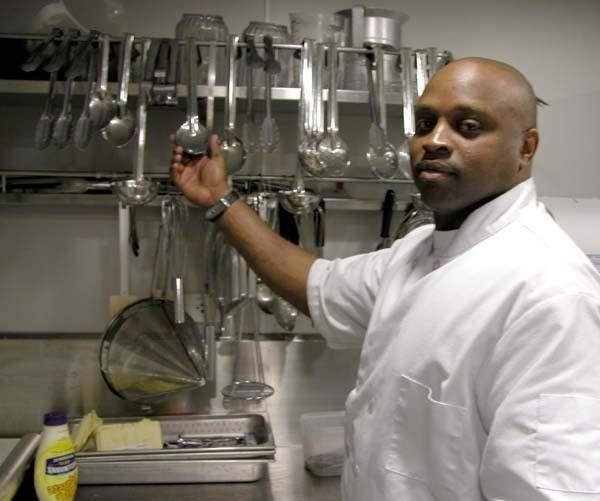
[167,57,600,501]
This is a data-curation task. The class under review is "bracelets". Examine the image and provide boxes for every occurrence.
[203,189,239,224]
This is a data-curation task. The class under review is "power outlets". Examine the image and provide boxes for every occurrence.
[183,293,223,324]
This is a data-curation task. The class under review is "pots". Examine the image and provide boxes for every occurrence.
[336,6,409,51]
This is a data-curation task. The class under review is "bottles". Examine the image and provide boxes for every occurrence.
[242,21,290,45]
[32,410,80,501]
[173,12,231,43]
